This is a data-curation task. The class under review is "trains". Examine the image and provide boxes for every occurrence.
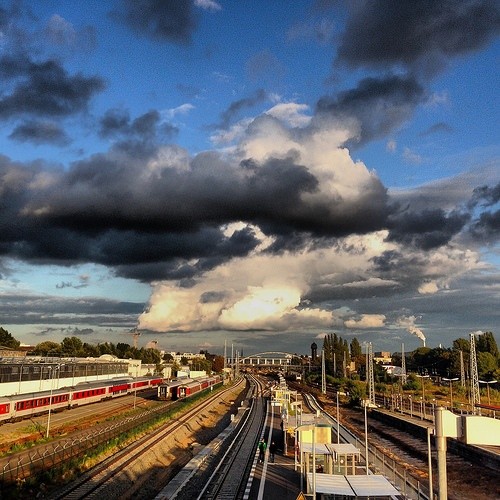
[157,374,221,401]
[0,377,164,426]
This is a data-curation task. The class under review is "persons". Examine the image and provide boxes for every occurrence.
[315,464,324,474]
[268,441,278,464]
[257,438,267,465]
[280,420,283,432]
